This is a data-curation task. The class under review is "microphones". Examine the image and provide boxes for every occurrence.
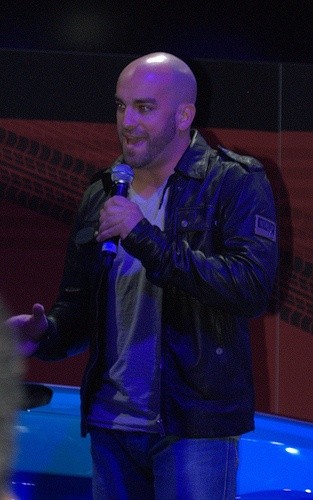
[102,164,134,269]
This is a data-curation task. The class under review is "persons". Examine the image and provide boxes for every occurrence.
[1,53,281,500]
